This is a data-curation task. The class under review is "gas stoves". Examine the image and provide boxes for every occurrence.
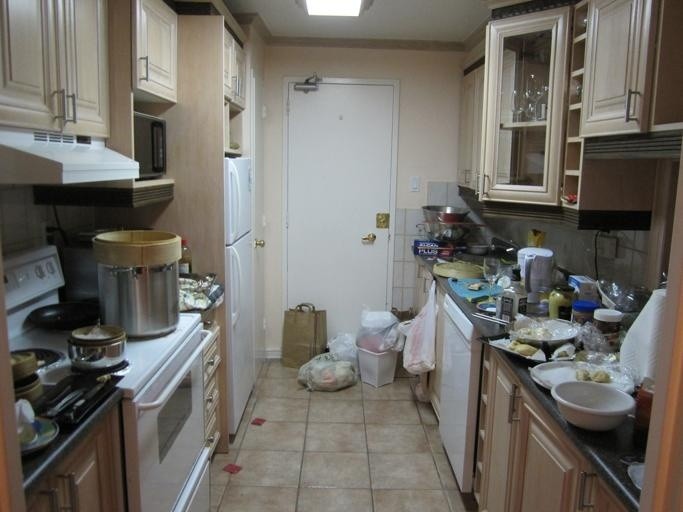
[7,312,201,400]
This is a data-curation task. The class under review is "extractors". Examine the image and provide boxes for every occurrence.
[0,125,141,185]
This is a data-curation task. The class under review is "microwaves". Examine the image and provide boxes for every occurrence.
[131,111,168,180]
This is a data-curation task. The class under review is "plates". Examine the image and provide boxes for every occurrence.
[28,375,124,423]
[19,417,60,458]
[530,361,635,395]
[477,302,497,314]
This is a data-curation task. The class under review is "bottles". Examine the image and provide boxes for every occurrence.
[591,308,623,349]
[571,299,599,329]
[178,241,192,277]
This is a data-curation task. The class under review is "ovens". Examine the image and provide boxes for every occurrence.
[124,322,214,512]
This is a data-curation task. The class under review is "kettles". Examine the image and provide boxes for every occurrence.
[516,246,556,306]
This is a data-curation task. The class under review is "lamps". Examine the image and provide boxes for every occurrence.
[290,1,374,19]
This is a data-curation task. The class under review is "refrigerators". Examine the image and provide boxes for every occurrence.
[223,157,258,436]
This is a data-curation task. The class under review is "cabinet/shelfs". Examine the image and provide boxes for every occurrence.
[574,461,641,511]
[410,254,448,422]
[473,336,587,512]
[197,314,230,465]
[456,0,683,233]
[131,1,177,104]
[0,1,111,138]
[223,27,251,158]
[25,399,125,511]
[133,93,176,209]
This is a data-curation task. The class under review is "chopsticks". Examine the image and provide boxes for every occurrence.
[471,312,509,326]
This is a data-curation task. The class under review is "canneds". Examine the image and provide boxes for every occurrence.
[548,284,622,352]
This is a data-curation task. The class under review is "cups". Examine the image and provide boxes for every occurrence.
[632,379,655,453]
[14,397,34,437]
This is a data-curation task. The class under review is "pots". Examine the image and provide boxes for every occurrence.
[68,326,123,369]
[415,222,481,242]
[26,301,88,330]
[93,262,180,338]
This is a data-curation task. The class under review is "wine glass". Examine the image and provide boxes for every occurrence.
[510,73,549,123]
[481,257,500,307]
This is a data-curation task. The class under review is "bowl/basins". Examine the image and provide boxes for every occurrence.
[421,205,470,222]
[469,244,489,255]
[503,316,578,348]
[549,380,637,431]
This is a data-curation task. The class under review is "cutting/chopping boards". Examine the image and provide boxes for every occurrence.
[447,276,502,300]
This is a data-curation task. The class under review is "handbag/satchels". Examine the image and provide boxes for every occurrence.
[280,309,327,369]
